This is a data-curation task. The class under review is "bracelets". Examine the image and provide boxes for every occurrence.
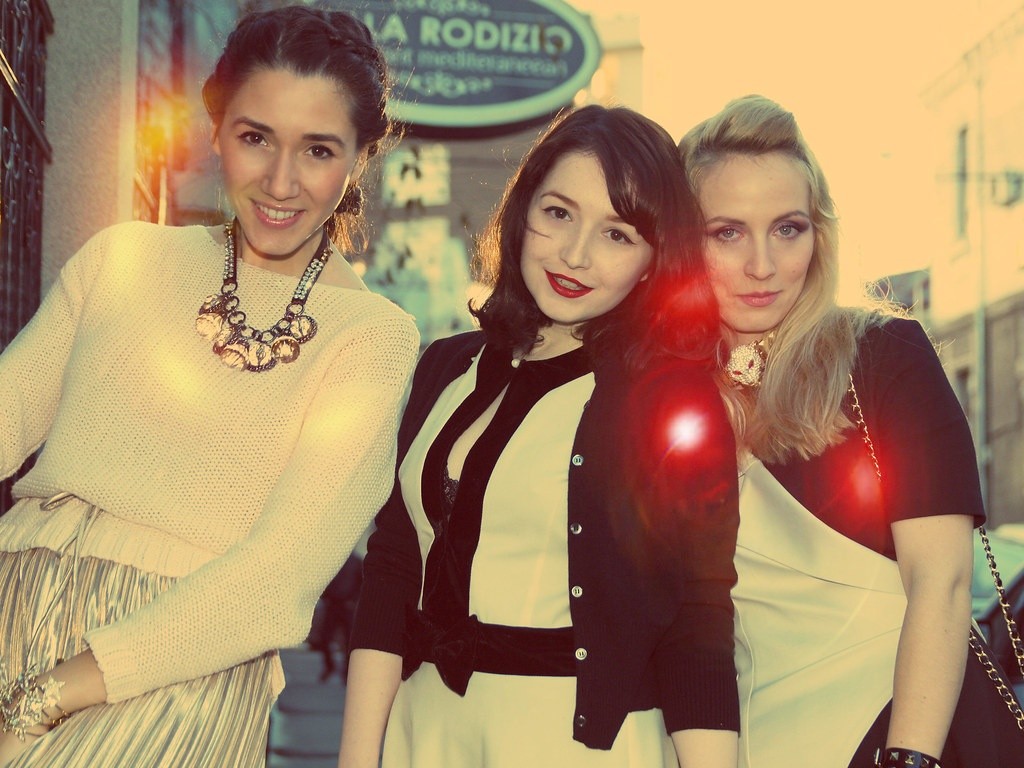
[0,661,71,742]
[883,748,942,768]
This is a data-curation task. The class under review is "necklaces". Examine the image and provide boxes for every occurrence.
[195,215,333,373]
[721,331,775,388]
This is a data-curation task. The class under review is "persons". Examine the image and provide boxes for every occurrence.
[339,106,740,768]
[0,5,422,768]
[677,94,1024,768]
